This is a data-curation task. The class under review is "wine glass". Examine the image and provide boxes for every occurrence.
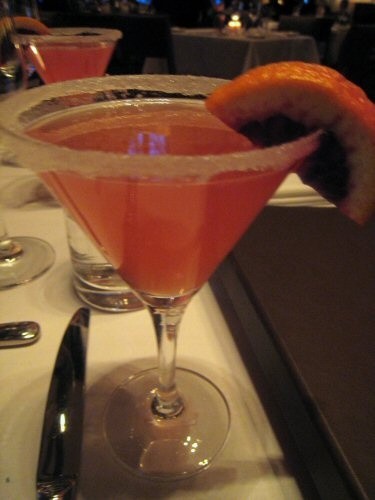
[0,74,322,479]
[10,26,123,84]
[0,208,55,289]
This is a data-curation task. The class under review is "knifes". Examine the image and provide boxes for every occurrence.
[36,307,90,499]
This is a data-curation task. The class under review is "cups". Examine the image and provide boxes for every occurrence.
[66,215,148,313]
